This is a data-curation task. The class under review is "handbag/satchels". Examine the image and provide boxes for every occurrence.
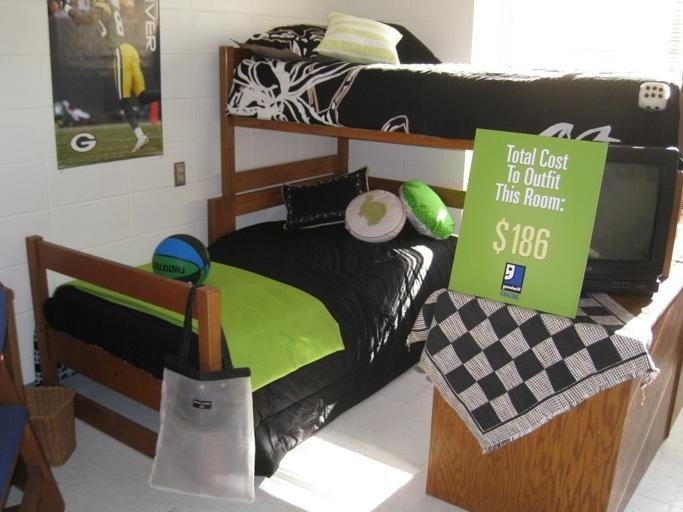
[148,283,256,503]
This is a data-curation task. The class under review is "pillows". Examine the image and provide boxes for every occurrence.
[231,25,327,59]
[281,167,369,230]
[399,181,454,240]
[345,189,405,243]
[386,23,441,64]
[313,13,403,65]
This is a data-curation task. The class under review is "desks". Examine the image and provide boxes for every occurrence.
[426,288,683,511]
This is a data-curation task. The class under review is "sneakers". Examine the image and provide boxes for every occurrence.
[130,135,149,153]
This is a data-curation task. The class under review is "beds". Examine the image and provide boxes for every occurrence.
[219,46,683,281]
[24,178,466,460]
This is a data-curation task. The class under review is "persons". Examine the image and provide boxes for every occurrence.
[49,0,160,151]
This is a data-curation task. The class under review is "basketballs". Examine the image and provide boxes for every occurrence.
[153,233,210,287]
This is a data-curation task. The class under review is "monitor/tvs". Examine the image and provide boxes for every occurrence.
[580,144,680,295]
